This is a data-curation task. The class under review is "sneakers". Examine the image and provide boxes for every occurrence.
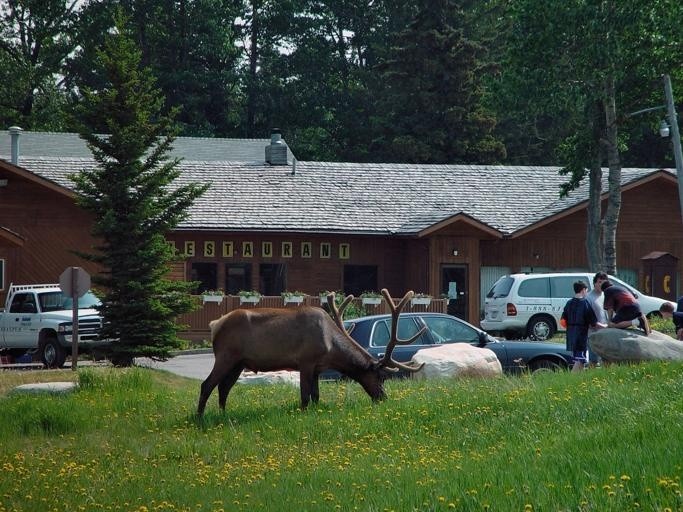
[638,311,651,335]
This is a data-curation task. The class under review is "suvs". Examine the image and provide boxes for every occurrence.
[475,269,676,344]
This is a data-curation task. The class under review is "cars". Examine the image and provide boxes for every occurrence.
[314,311,578,387]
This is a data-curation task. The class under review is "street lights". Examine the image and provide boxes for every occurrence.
[626,99,682,216]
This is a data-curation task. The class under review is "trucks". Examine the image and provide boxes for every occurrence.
[0,278,133,370]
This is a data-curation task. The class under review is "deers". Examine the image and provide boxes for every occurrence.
[196,287,427,417]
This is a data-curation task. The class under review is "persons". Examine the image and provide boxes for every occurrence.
[558,280,609,375]
[585,269,611,368]
[600,277,649,336]
[659,302,682,341]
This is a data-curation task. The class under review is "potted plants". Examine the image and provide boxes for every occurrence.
[199,290,432,309]
